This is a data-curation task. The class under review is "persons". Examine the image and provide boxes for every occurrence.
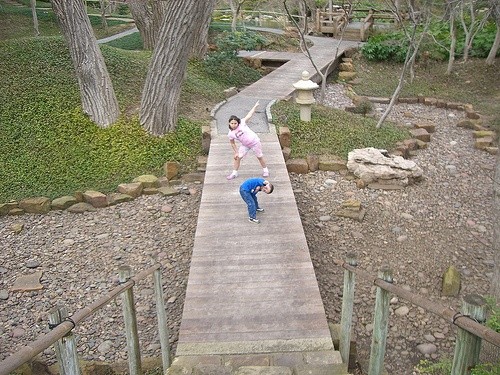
[240,177,273,224]
[226,99,269,179]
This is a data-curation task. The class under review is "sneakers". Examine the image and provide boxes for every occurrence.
[256,208,264,213]
[263,170,269,177]
[227,173,238,180]
[248,217,260,225]
